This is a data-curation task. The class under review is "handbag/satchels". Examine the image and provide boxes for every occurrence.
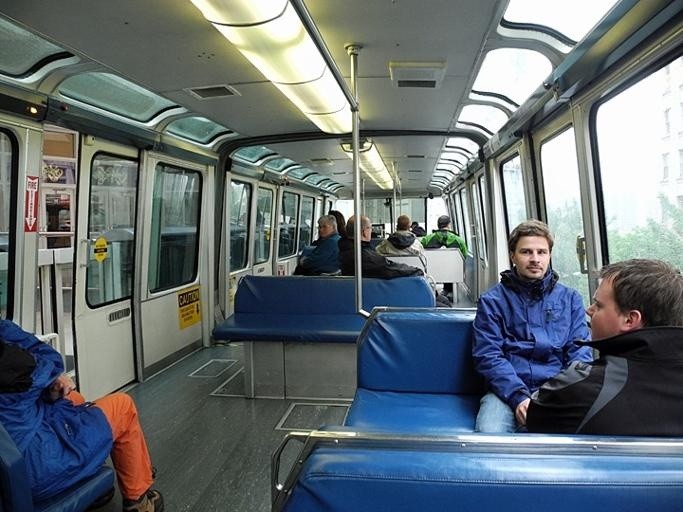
[40,388,62,403]
[303,246,317,256]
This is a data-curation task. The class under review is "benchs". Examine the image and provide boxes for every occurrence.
[267,425,683,512]
[386,248,466,306]
[342,306,490,434]
[212,275,437,401]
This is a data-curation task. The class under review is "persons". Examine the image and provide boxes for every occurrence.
[1,318,165,512]
[472,217,595,434]
[294,211,468,308]
[523,258,682,438]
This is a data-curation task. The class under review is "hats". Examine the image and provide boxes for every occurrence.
[410,222,418,228]
[438,216,450,224]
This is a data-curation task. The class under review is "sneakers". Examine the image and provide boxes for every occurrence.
[123,488,163,512]
